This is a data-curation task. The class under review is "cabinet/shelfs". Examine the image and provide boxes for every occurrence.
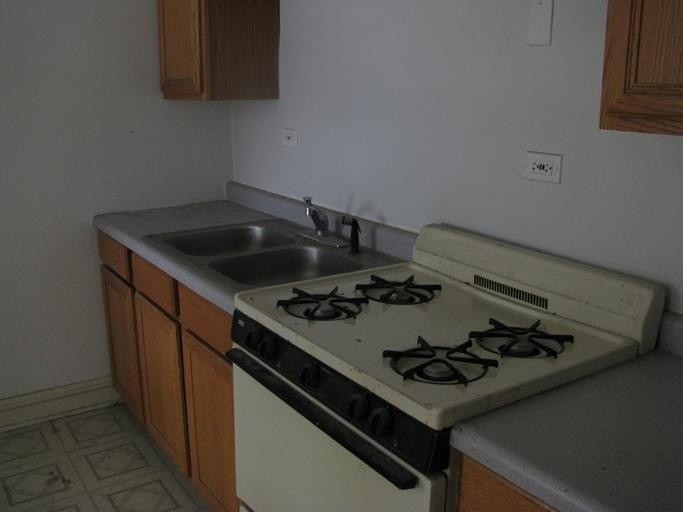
[156,1,279,101]
[598,0,683,136]
[94,229,189,478]
[174,283,237,510]
[446,449,541,512]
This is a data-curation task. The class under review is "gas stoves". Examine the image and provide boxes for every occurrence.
[234,260,641,474]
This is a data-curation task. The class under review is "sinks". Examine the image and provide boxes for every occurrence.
[207,244,365,298]
[141,224,296,257]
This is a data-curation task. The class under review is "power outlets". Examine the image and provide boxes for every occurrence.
[279,129,297,147]
[524,151,564,184]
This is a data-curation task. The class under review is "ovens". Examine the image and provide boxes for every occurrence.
[226,348,438,511]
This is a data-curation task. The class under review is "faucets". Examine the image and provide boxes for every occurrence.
[303,196,329,235]
